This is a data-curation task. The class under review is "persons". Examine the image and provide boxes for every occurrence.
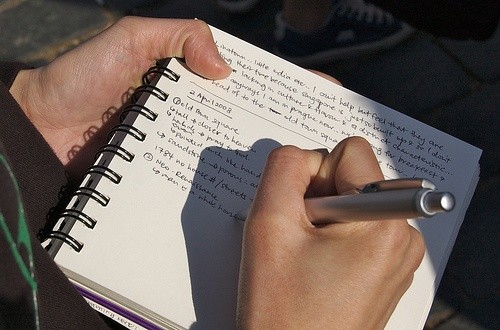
[217,0,410,65]
[1,17,426,330]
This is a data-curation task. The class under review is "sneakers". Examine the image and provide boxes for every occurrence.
[272,0,411,68]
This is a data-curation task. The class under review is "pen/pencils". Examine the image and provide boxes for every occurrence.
[232,178,457,228]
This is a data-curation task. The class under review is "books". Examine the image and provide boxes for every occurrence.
[40,16,482,329]
